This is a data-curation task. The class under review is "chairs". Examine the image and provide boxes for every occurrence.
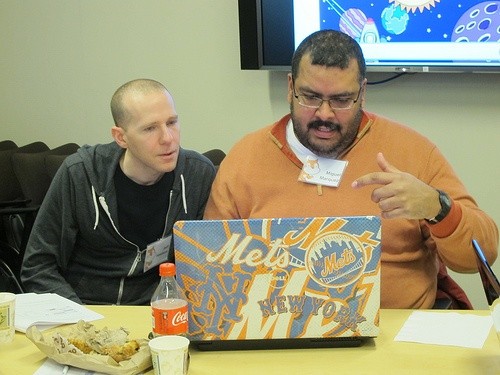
[0,140,225,293]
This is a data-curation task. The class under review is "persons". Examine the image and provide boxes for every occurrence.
[203,30,500,311]
[20,80,218,305]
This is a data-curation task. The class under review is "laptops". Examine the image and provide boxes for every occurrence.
[173,216,382,349]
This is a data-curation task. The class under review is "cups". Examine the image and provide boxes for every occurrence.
[0,292,16,345]
[148,335,191,375]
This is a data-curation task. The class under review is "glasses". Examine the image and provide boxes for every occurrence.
[292,73,362,110]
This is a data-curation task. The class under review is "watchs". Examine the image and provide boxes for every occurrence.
[425,189,453,225]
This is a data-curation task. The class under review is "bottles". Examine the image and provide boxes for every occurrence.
[150,262,189,338]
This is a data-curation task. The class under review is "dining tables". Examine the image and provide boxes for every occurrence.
[0,302,500,375]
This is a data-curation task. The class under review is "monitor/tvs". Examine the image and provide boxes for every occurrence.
[238,0,500,75]
